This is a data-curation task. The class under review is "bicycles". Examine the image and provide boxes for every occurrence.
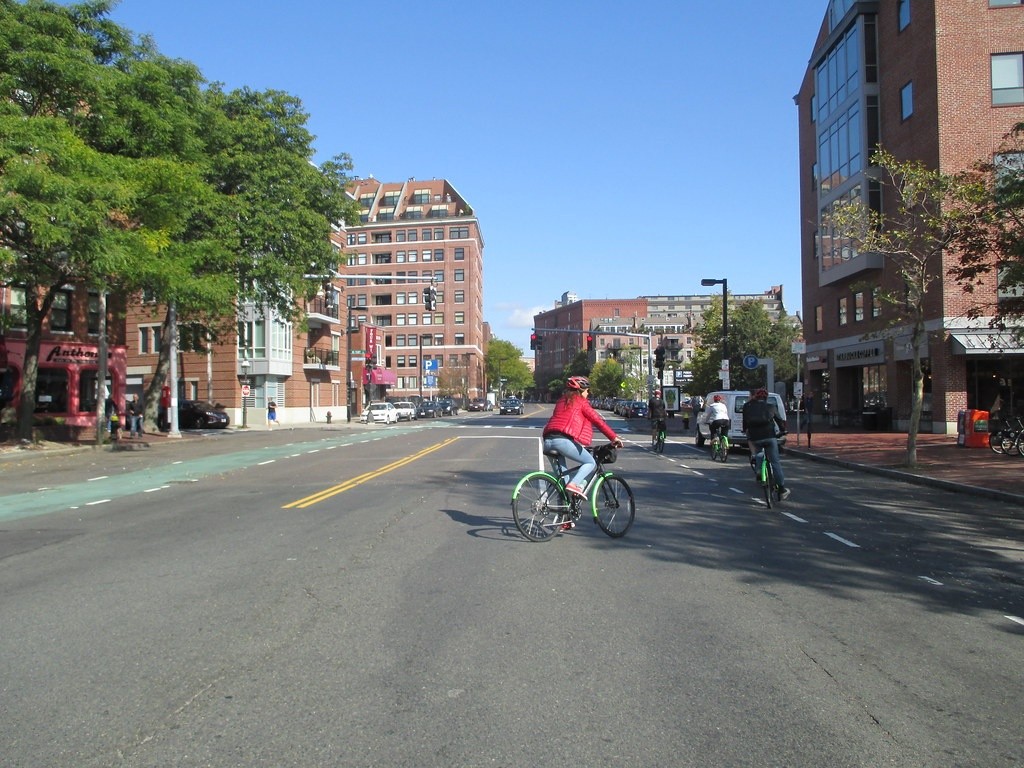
[510,438,635,542]
[649,416,665,452]
[741,431,789,509]
[703,419,729,463]
[989,415,1024,456]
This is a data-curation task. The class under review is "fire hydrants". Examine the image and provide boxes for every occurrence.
[326,411,332,424]
[683,413,691,430]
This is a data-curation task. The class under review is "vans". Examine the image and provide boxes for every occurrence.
[695,390,788,450]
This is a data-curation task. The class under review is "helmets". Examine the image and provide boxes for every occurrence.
[755,388,769,399]
[713,395,721,400]
[654,389,663,395]
[567,376,592,390]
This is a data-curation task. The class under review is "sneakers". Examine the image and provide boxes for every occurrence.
[564,482,589,502]
[779,488,791,500]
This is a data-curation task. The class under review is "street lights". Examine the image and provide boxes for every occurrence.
[347,307,368,419]
[241,359,250,427]
[701,278,730,390]
[419,334,432,398]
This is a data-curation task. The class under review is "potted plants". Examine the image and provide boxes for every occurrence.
[327,352,332,366]
[307,351,316,363]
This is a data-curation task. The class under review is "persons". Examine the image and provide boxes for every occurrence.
[266,397,280,431]
[691,396,700,412]
[703,395,730,448]
[742,388,790,501]
[647,390,667,446]
[129,393,143,439]
[799,391,814,433]
[105,394,119,432]
[542,376,624,532]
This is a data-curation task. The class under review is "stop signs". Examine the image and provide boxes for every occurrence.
[242,385,250,397]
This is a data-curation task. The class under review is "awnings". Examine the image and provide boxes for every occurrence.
[362,365,396,385]
[951,328,1024,356]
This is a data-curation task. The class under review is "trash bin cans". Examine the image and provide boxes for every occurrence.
[161,386,170,408]
[862,412,877,431]
[957,410,989,448]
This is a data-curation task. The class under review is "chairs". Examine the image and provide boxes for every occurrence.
[506,401,510,404]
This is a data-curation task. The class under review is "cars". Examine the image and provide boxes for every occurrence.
[360,399,493,425]
[500,400,524,415]
[588,398,649,418]
[864,396,884,407]
[159,400,230,430]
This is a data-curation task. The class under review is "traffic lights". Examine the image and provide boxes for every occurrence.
[531,334,536,350]
[365,353,371,370]
[587,336,593,351]
[424,286,431,311]
